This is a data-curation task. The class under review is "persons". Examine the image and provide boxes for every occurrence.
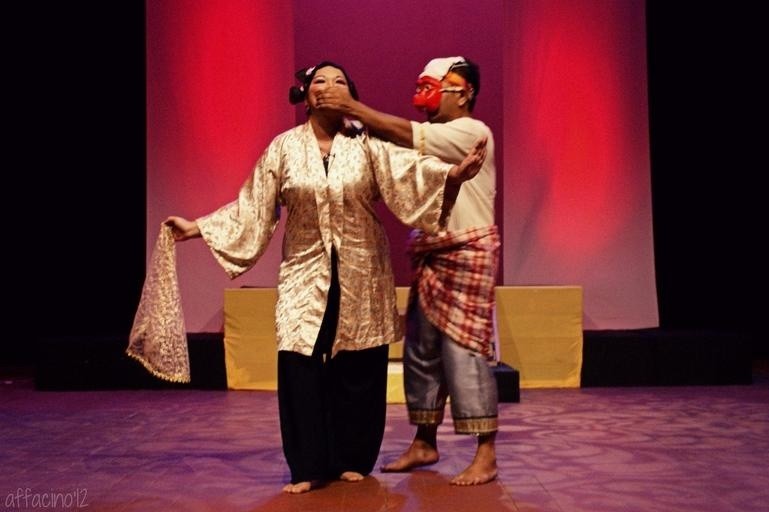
[164,62,488,493]
[315,53,501,486]
